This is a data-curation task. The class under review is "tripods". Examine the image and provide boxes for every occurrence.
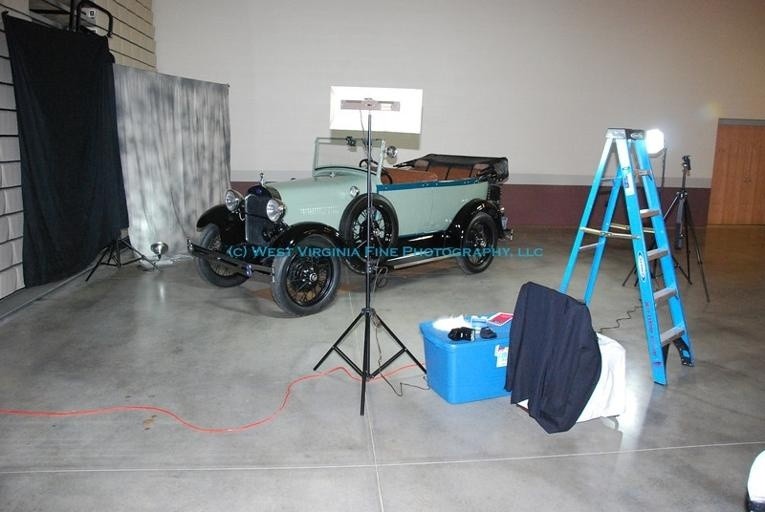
[314,115,427,415]
[86,237,161,281]
[622,155,709,302]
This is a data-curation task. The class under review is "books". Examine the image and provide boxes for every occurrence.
[486,312,514,326]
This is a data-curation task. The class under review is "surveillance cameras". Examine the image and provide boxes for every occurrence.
[89,10,96,18]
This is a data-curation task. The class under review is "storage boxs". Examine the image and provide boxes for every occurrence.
[421,312,514,406]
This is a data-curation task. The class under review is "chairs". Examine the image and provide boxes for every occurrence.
[371,160,490,186]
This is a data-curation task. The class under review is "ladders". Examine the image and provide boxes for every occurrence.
[558,127,693,386]
